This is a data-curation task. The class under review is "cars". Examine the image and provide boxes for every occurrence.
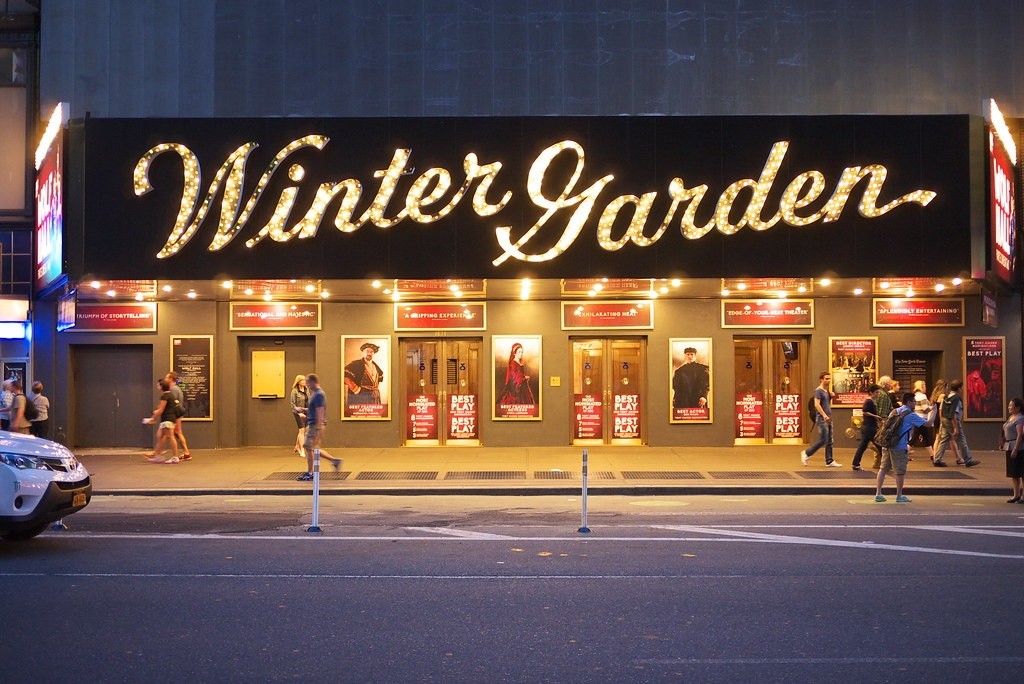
[0,429,91,541]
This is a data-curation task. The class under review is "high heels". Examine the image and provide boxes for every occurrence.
[1018,500,1024,503]
[1007,496,1020,503]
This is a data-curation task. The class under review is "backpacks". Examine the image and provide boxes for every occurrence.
[13,394,39,420]
[874,409,914,448]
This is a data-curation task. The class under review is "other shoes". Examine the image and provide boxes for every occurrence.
[297,472,314,481]
[957,458,964,464]
[934,462,947,467]
[333,459,341,473]
[294,446,300,452]
[966,460,980,467]
[299,448,306,457]
[852,464,864,470]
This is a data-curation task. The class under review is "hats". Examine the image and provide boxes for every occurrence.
[360,343,378,353]
[305,374,319,382]
[684,347,697,354]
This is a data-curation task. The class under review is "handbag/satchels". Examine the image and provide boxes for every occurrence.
[808,390,831,423]
[998,430,1005,446]
[174,404,186,419]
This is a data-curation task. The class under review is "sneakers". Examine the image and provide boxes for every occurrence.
[148,455,165,462]
[165,457,180,463]
[179,453,192,461]
[875,496,887,501]
[144,454,155,458]
[826,460,842,467]
[896,496,912,502]
[801,451,808,465]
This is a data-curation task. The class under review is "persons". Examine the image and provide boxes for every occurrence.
[344,343,383,404]
[851,375,981,472]
[875,393,938,503]
[673,347,709,408]
[495,343,538,404]
[141,372,193,464]
[0,379,50,440]
[290,373,343,481]
[999,398,1024,504]
[801,372,843,468]
[832,353,875,368]
